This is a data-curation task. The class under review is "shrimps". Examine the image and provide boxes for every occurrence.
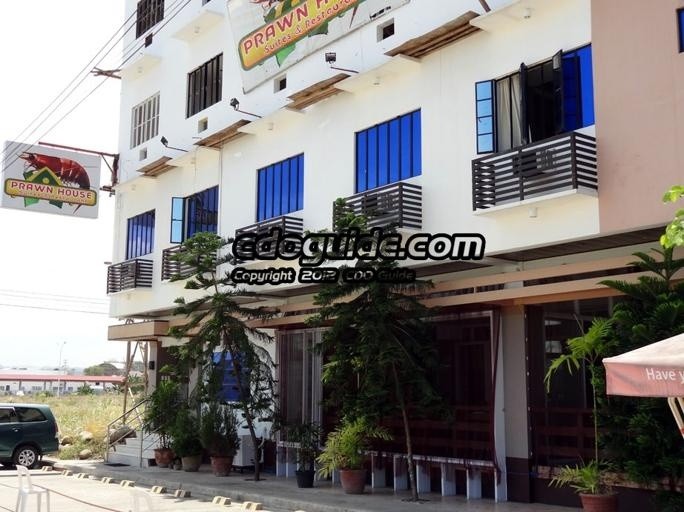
[14,150,91,215]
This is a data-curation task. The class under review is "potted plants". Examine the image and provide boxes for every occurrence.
[273,419,323,487]
[135,380,241,479]
[315,414,388,495]
[538,309,628,512]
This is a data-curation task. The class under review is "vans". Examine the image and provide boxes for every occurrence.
[0,403,61,466]
